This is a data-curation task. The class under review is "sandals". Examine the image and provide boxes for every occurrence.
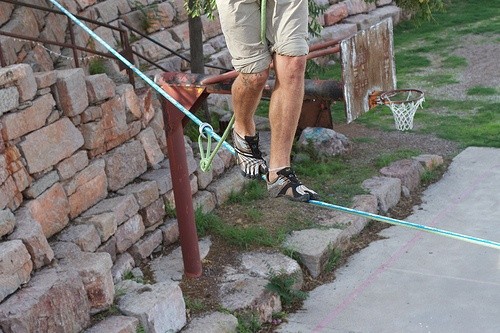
[264,167,318,202]
[230,123,269,180]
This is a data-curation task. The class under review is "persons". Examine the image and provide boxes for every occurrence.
[216,0,320,201]
